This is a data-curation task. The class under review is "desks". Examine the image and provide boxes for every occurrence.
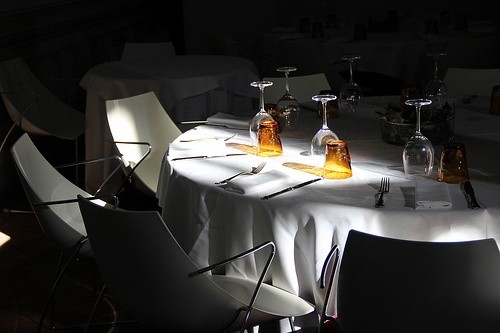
[76,57,256,200]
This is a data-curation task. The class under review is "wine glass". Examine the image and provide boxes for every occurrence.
[310,94,339,158]
[426,52,447,105]
[340,55,361,111]
[249,81,274,140]
[402,99,435,176]
[274,67,300,130]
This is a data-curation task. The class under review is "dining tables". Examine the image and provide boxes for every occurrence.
[268,23,496,93]
[154,94,500,333]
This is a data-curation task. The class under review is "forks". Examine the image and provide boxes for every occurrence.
[181,131,238,142]
[214,161,267,185]
[378,177,390,208]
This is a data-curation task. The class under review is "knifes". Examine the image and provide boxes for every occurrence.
[265,178,321,199]
[173,154,247,160]
[464,180,480,210]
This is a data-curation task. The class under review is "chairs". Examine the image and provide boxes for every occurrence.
[439,68,500,96]
[261,72,332,108]
[312,231,500,333]
[122,42,176,59]
[0,55,86,186]
[101,91,182,195]
[10,132,127,333]
[76,188,318,333]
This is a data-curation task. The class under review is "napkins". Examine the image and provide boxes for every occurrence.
[279,32,303,40]
[207,112,251,128]
[414,179,452,210]
[272,27,295,33]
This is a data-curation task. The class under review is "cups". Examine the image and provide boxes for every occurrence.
[438,141,470,183]
[317,90,340,118]
[258,122,282,156]
[321,140,352,179]
[488,85,500,114]
[265,104,282,133]
